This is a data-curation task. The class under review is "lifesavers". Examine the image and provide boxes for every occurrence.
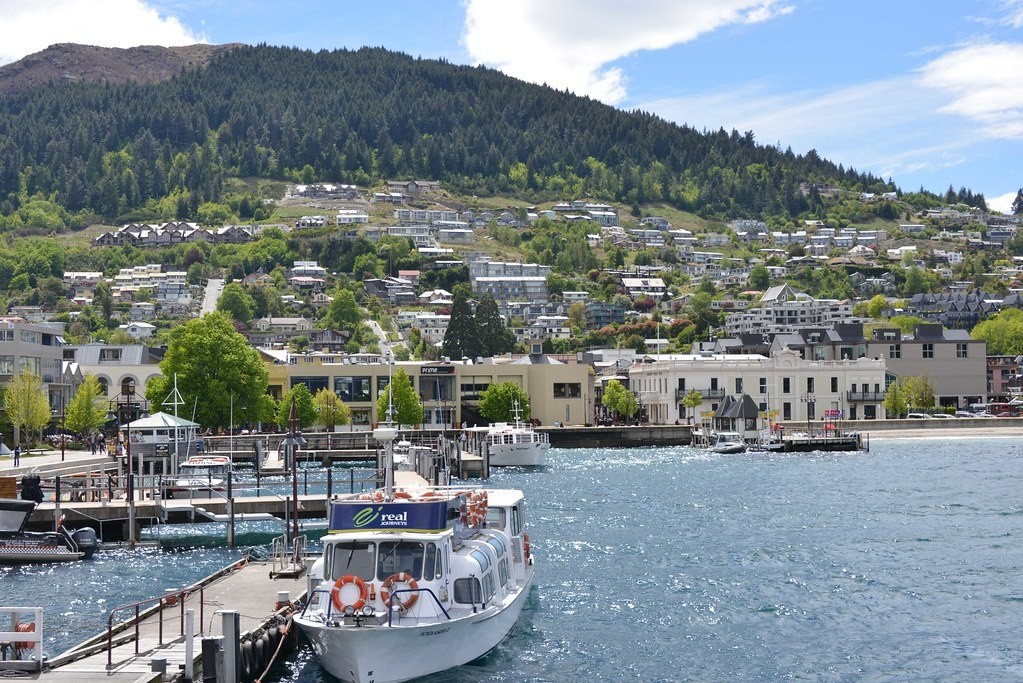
[189,457,203,462]
[369,492,444,503]
[332,575,368,612]
[57,517,62,532]
[213,457,228,462]
[523,533,530,568]
[379,572,419,610]
[455,491,488,527]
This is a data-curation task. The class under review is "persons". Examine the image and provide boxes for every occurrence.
[206,428,213,435]
[85,431,106,455]
[675,418,680,425]
[555,420,564,427]
[252,426,257,435]
[14,442,21,467]
[461,421,467,429]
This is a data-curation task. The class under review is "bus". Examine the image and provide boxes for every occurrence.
[969,404,986,413]
[986,404,1023,415]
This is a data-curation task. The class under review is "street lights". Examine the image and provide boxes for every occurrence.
[450,405,456,428]
[637,398,641,424]
[51,404,65,461]
[332,402,338,431]
[326,403,332,431]
[1008,392,1011,418]
[419,395,425,430]
[763,395,770,409]
[919,394,924,418]
[801,391,816,445]
[838,397,844,420]
[104,400,153,503]
[584,398,593,426]
[907,404,910,420]
[385,347,395,427]
[442,399,446,431]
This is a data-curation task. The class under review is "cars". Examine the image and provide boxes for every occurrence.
[933,414,955,419]
[954,412,978,417]
[978,411,1023,417]
[906,413,932,419]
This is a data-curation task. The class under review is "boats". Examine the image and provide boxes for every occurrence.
[169,455,242,500]
[709,432,747,453]
[387,436,422,471]
[49,472,117,499]
[292,352,535,683]
[0,498,99,564]
[479,398,552,468]
